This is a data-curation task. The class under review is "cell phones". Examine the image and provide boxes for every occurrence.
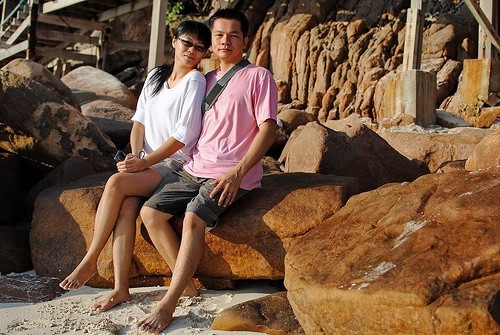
[114,150,126,162]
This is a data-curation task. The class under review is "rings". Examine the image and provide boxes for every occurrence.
[227,192,232,196]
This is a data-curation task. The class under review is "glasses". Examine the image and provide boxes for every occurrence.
[177,36,207,53]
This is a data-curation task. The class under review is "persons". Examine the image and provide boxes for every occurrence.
[59,20,212,314]
[134,8,278,334]
